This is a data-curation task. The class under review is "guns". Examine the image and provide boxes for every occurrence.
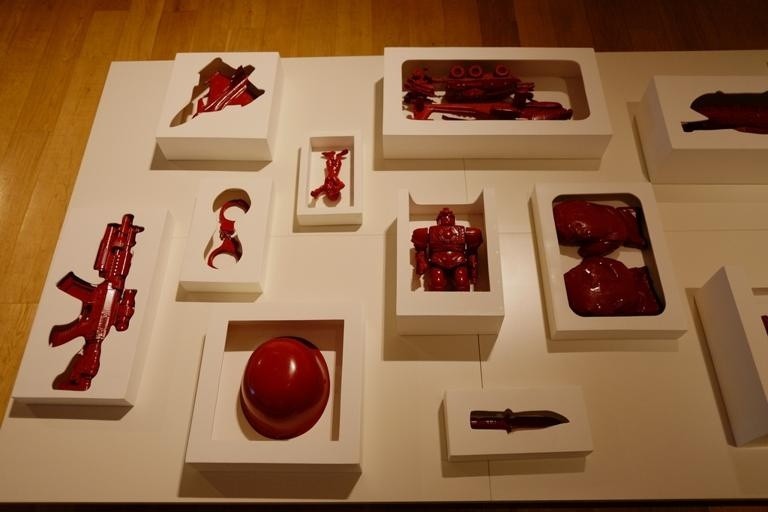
[45,209,146,397]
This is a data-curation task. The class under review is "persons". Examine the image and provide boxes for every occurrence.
[310,145,349,204]
[410,207,486,292]
